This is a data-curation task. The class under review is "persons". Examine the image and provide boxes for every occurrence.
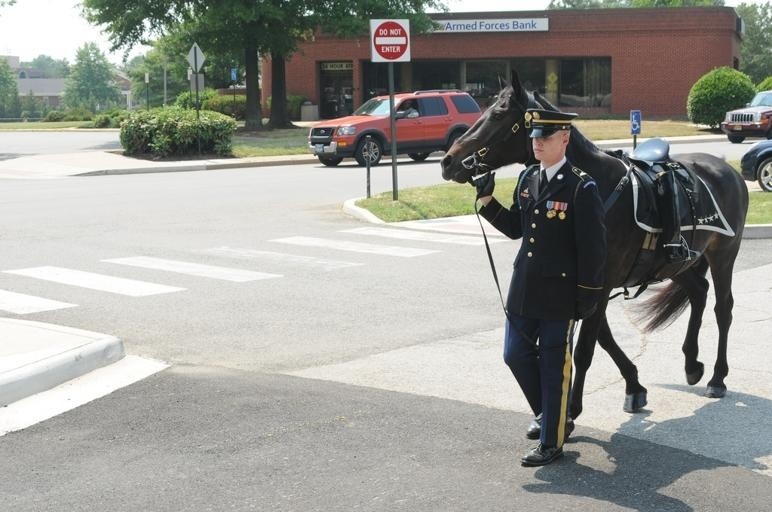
[404,100,419,118]
[474,108,602,465]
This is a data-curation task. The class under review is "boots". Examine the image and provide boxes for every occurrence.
[655,170,701,263]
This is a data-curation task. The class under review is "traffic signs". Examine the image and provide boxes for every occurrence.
[144,72,151,113]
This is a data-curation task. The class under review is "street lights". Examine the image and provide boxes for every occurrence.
[187,65,194,109]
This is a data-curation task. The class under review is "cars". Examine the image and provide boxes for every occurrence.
[739,140,772,194]
[720,89,772,144]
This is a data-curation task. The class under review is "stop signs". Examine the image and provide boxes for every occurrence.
[369,17,411,65]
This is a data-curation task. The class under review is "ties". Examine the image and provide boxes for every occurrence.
[539,169,548,194]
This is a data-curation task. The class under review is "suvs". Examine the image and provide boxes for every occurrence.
[307,88,484,168]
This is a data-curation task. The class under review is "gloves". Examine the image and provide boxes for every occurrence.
[474,172,495,198]
[575,298,598,321]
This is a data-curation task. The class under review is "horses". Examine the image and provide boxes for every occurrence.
[438,65,751,441]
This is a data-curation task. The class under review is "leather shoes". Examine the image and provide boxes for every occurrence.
[520,443,563,465]
[526,412,543,439]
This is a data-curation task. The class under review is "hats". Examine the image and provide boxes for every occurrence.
[526,109,579,138]
